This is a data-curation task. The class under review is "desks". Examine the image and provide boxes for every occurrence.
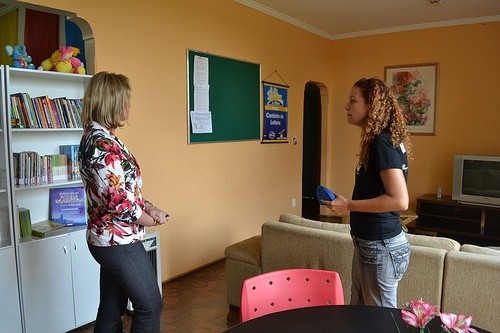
[223,305,493,333]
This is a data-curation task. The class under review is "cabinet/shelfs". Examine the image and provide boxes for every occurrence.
[415,193,500,236]
[0,65,100,333]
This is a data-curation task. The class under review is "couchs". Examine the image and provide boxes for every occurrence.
[225,213,500,333]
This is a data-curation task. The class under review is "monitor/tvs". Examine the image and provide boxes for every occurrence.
[451,155,500,208]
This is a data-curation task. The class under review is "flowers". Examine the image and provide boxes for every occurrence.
[401,297,479,333]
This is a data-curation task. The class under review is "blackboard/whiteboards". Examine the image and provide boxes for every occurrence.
[185,47,264,145]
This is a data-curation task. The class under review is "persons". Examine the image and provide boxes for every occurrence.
[320,77,413,309]
[77,71,171,333]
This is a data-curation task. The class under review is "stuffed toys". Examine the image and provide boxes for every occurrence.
[5,44,35,70]
[38,45,85,75]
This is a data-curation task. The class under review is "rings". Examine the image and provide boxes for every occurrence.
[165,214,169,217]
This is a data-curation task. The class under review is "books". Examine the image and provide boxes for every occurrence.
[10,93,85,128]
[17,187,87,238]
[13,145,80,185]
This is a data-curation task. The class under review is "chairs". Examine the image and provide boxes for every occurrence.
[238,268,345,323]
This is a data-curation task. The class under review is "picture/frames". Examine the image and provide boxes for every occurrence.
[383,62,439,137]
